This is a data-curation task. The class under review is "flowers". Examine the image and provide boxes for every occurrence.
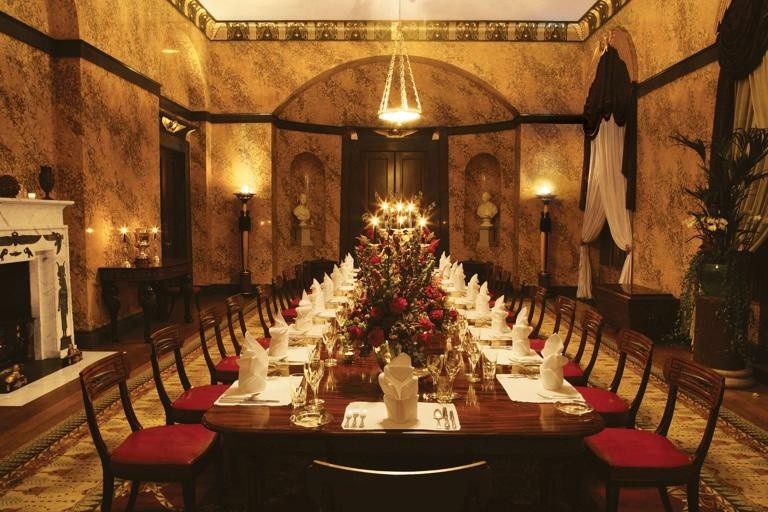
[341,224,458,366]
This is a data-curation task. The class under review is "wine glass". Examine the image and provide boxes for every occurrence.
[424,310,481,402]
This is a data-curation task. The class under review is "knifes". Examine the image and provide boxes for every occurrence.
[442,406,456,429]
[219,399,280,404]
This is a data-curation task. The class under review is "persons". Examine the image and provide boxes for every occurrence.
[293,191,311,222]
[477,191,498,220]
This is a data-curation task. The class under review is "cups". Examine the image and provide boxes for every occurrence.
[295,316,312,330]
[474,300,490,315]
[539,365,565,391]
[302,269,362,405]
[236,366,268,393]
[382,395,420,426]
[482,353,498,380]
[269,336,289,357]
[490,318,508,331]
[512,337,532,357]
[289,374,309,407]
[453,278,465,290]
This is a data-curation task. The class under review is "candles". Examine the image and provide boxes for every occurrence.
[123,229,127,242]
[154,233,157,239]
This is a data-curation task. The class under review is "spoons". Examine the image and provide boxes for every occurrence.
[221,391,261,400]
[433,408,442,430]
[344,406,367,429]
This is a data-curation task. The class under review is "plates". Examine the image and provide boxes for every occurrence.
[291,410,335,428]
[553,400,594,417]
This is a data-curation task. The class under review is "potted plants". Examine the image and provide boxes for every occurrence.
[669,125,768,296]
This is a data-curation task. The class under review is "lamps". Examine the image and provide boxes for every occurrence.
[232,186,257,293]
[377,6,422,123]
[537,187,560,287]
